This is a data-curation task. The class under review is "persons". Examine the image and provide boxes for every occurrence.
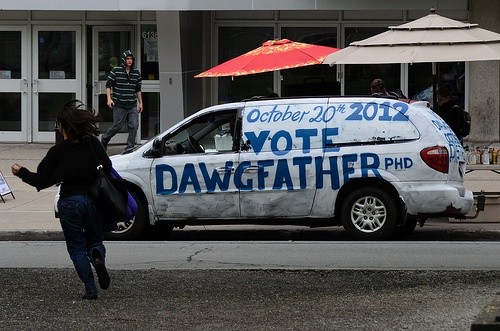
[11,98,114,301]
[370,79,390,96]
[101,50,144,152]
[435,87,466,147]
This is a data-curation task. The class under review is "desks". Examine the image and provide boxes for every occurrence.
[466,164,500,174]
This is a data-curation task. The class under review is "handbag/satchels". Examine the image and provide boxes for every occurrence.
[86,138,128,222]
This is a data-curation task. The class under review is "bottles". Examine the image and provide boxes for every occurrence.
[463,143,500,165]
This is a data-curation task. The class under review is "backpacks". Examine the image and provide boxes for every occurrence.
[449,104,471,137]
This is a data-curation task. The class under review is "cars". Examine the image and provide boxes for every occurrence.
[54,94,474,242]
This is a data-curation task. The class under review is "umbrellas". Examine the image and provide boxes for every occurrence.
[193,38,341,97]
[322,7,500,113]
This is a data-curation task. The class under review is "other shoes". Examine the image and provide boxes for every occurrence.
[94,257,110,290]
[101,136,107,150]
[82,292,98,299]
